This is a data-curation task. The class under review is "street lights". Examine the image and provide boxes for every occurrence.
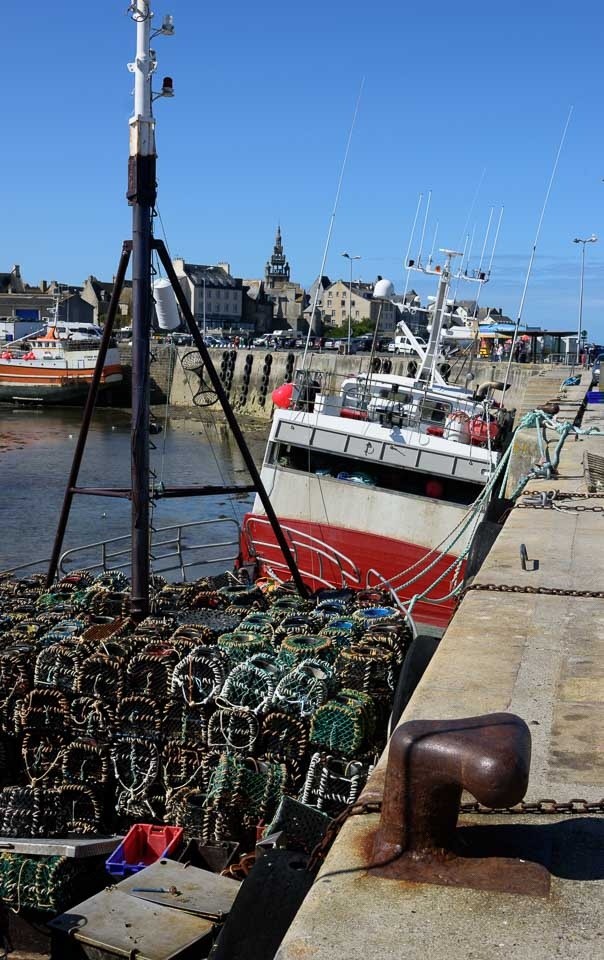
[573,236,597,363]
[340,251,362,354]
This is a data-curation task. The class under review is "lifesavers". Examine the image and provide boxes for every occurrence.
[238,354,253,405]
[382,360,391,374]
[283,354,296,382]
[339,408,368,421]
[258,354,272,405]
[426,426,444,439]
[406,360,417,378]
[219,349,236,402]
[371,358,381,373]
[440,363,451,384]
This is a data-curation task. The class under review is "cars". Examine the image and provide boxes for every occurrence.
[202,334,427,356]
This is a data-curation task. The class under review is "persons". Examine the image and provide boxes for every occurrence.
[158,332,325,351]
[491,343,527,363]
[293,369,321,413]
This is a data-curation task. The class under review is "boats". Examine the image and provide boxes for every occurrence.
[242,78,573,643]
[1,291,123,403]
[0,0,422,960]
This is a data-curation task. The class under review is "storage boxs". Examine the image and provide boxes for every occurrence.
[105,824,240,884]
